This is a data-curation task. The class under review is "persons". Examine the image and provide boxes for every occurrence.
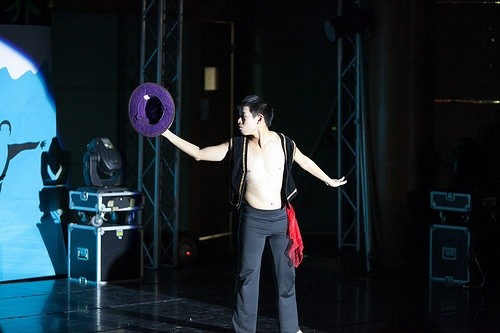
[160,94,348,332]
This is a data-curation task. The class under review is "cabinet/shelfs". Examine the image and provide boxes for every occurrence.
[66,186,144,287]
[429,191,496,289]
